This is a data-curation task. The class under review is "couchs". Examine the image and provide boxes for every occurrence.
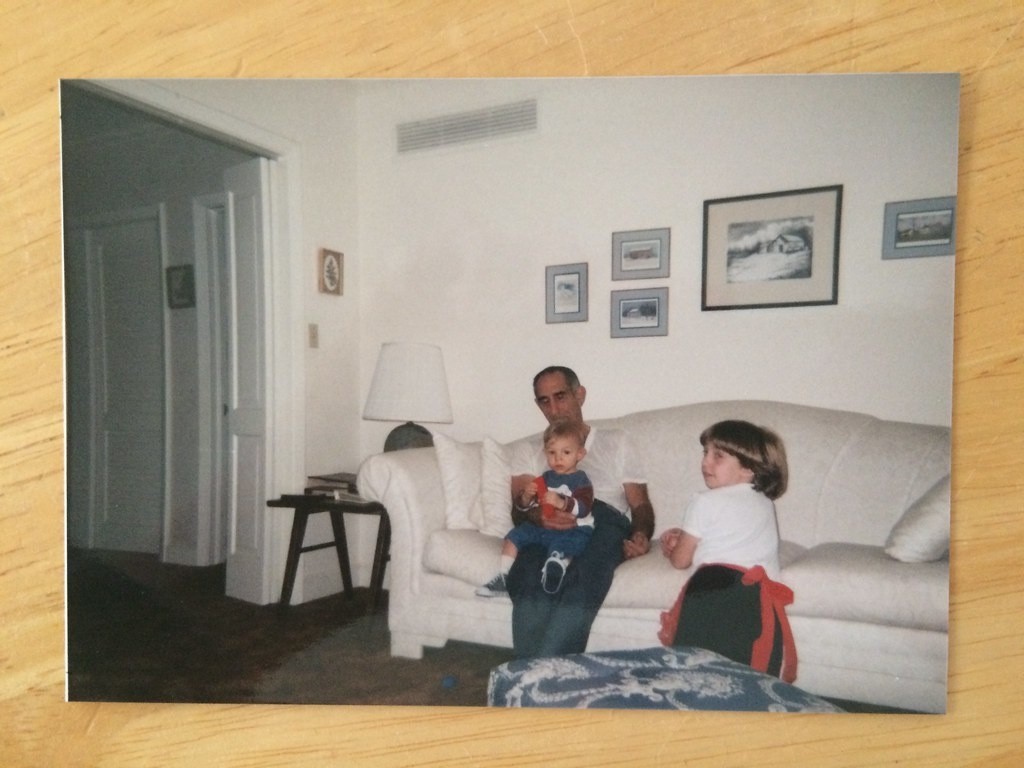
[355,398,951,714]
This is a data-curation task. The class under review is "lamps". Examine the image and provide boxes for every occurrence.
[361,336,454,453]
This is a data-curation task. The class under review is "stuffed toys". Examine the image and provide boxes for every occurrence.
[510,473,577,531]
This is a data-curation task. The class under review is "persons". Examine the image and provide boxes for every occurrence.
[657,419,798,684]
[507,366,655,656]
[476,422,593,598]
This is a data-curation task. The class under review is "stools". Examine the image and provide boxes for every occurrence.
[487,644,844,714]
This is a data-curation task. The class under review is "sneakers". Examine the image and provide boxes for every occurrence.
[476,573,509,597]
[541,551,566,593]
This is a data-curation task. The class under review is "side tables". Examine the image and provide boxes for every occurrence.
[266,497,391,641]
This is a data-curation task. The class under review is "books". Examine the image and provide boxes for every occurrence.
[307,472,356,487]
[304,485,358,500]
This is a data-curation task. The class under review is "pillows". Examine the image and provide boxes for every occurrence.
[432,430,482,532]
[476,435,515,538]
[884,471,951,565]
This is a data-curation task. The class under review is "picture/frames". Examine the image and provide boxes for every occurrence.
[318,246,345,296]
[545,262,589,324]
[701,184,843,312]
[610,286,669,339]
[165,263,196,309]
[611,227,671,281]
[880,194,956,260]
